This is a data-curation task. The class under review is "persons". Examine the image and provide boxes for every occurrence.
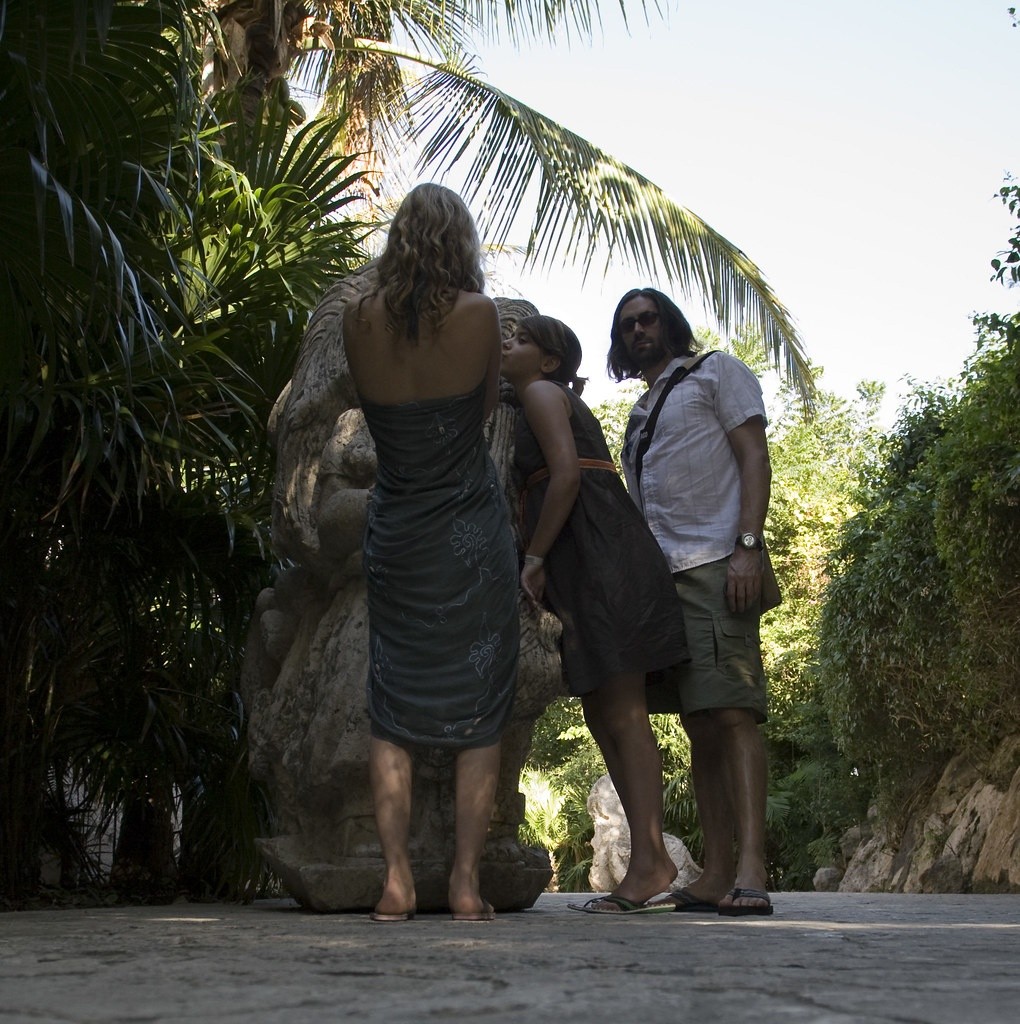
[502,316,680,914]
[608,289,782,914]
[342,183,521,921]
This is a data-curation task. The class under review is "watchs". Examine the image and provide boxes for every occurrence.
[734,533,762,550]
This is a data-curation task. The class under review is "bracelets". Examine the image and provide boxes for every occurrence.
[524,555,543,566]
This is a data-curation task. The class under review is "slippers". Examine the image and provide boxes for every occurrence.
[648,888,720,912]
[567,893,677,913]
[370,909,416,921]
[452,896,496,920]
[719,887,774,916]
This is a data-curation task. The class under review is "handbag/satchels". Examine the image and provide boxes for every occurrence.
[761,536,781,614]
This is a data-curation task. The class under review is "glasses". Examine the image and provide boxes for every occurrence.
[619,311,660,334]
